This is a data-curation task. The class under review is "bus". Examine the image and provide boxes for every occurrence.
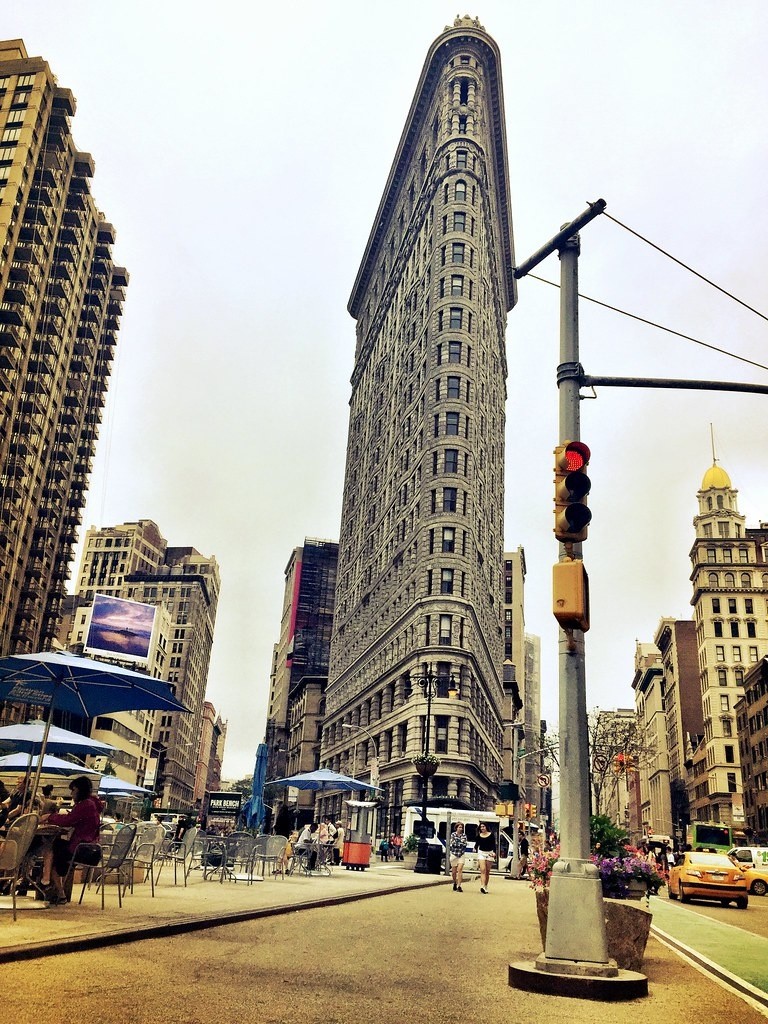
[404,806,543,873]
[686,821,732,852]
[149,813,188,824]
[637,835,674,856]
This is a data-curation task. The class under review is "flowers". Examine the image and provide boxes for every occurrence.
[411,753,441,764]
[525,814,668,898]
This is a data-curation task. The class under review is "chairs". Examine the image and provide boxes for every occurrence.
[0,813,336,921]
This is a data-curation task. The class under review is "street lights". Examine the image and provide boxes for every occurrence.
[655,811,682,851]
[342,724,379,848]
[130,740,193,807]
[278,749,301,830]
[502,722,526,879]
[400,663,458,874]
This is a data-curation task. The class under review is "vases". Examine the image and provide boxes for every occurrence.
[416,763,438,777]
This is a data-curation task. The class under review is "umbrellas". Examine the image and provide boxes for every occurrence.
[0,643,195,874]
[99,772,157,821]
[0,720,123,817]
[240,743,268,829]
[0,753,111,778]
[98,790,133,797]
[263,768,385,859]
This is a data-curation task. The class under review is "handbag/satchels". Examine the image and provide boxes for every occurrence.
[74,840,102,866]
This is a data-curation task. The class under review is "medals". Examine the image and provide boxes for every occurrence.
[104,810,121,822]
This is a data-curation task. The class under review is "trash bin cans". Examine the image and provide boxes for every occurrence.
[341,840,371,871]
[426,844,442,875]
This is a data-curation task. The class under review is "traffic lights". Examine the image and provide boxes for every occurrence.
[624,753,635,773]
[524,804,529,819]
[647,826,651,835]
[553,439,592,543]
[613,753,625,773]
[552,556,591,632]
[531,805,537,820]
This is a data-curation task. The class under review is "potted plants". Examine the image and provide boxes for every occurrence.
[401,833,420,869]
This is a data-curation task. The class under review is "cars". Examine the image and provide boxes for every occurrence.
[666,847,768,909]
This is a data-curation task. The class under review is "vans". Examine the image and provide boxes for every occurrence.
[727,847,768,870]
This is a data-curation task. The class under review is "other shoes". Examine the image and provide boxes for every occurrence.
[36,893,68,905]
[481,888,488,894]
[453,884,457,891]
[27,880,52,891]
[272,870,281,874]
[457,887,462,892]
[285,869,290,874]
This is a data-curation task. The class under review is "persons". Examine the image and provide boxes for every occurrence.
[637,842,674,869]
[474,822,496,894]
[450,823,468,892]
[295,816,344,871]
[0,775,104,903]
[271,805,292,874]
[157,816,163,827]
[379,834,404,862]
[544,840,552,852]
[515,831,531,880]
[173,818,224,848]
